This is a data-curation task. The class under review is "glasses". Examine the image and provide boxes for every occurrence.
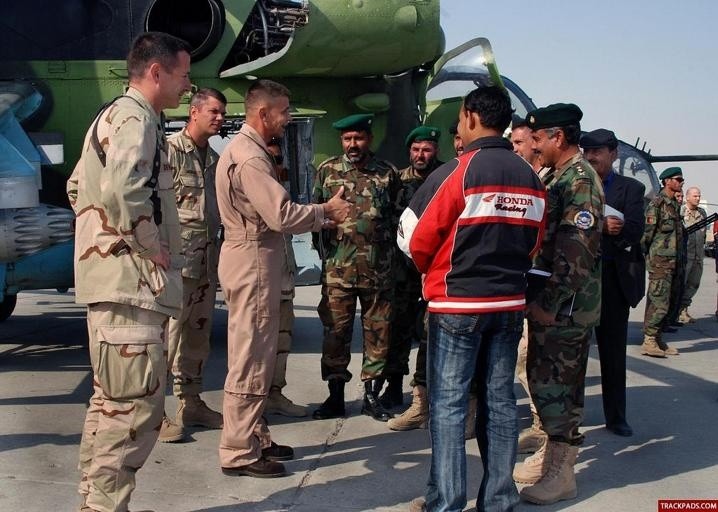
[669,177,684,184]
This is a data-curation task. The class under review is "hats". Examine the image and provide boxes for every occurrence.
[578,128,619,151]
[659,166,683,181]
[511,114,530,130]
[523,103,584,131]
[447,118,461,134]
[403,125,442,150]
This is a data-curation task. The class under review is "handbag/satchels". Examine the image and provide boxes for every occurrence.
[614,234,647,309]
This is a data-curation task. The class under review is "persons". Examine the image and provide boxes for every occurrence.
[507,100,650,507]
[658,186,686,334]
[262,140,310,420]
[214,79,355,481]
[393,84,546,512]
[310,111,400,424]
[639,166,682,358]
[379,123,446,412]
[156,85,226,446]
[383,117,478,444]
[62,28,194,512]
[678,186,708,325]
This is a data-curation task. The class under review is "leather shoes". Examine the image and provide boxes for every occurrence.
[222,457,287,478]
[261,440,295,462]
[605,419,634,437]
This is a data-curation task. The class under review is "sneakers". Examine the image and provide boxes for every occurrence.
[407,497,429,512]
[677,308,697,324]
[261,388,308,418]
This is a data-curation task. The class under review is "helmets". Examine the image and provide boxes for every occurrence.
[332,113,375,132]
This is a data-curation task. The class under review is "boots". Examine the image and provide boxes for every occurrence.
[387,384,430,432]
[311,379,348,420]
[515,410,548,455]
[655,335,679,355]
[359,379,393,424]
[464,396,478,440]
[511,436,555,485]
[378,376,404,410]
[156,410,187,443]
[639,333,666,357]
[519,441,578,505]
[175,392,225,429]
